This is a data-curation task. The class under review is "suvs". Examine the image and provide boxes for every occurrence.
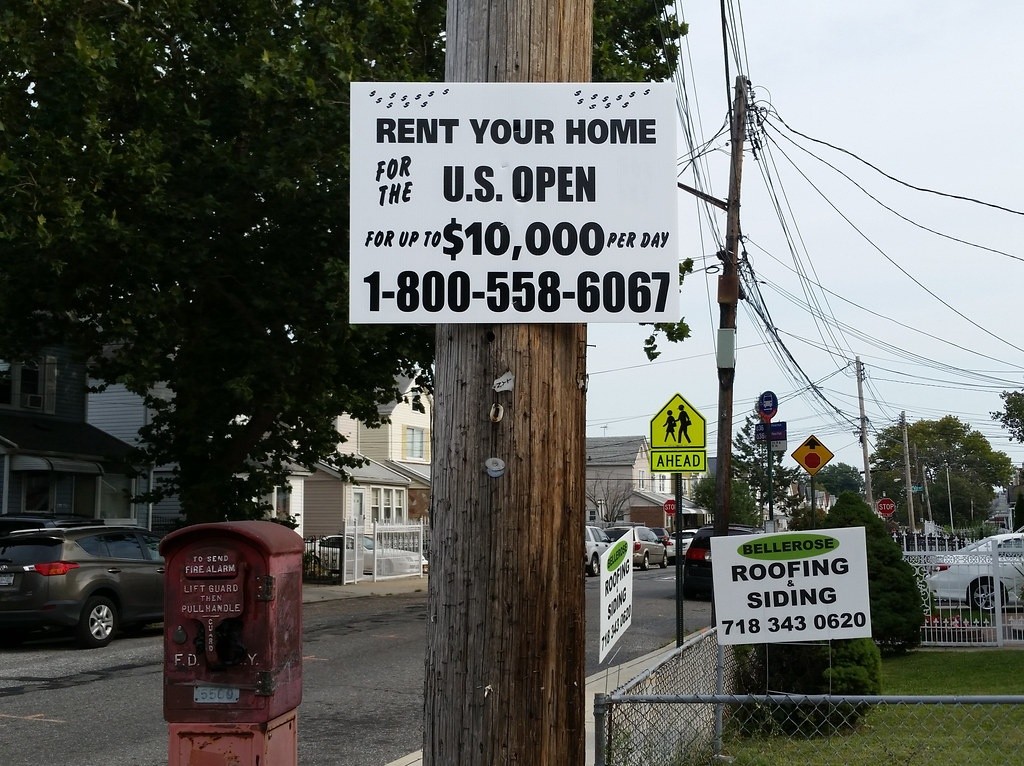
[1,515,168,651]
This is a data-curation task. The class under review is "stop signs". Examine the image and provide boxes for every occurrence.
[875,498,897,518]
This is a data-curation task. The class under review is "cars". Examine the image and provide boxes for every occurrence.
[586,521,767,604]
[923,533,1023,613]
[301,533,427,576]
